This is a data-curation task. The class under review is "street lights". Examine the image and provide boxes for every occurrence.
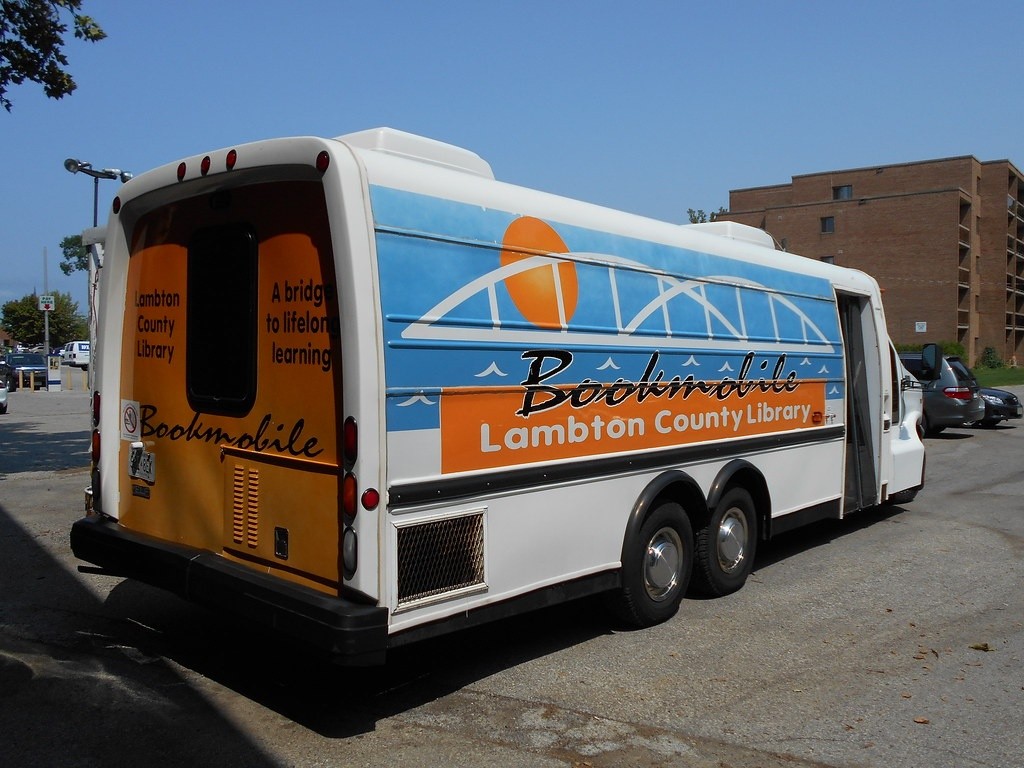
[62,158,134,454]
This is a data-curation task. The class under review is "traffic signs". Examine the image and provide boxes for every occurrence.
[39,296,56,310]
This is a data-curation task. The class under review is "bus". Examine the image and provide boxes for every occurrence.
[65,126,943,658]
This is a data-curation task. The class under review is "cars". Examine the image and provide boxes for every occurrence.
[0,343,63,393]
[0,360,9,414]
[961,385,1024,428]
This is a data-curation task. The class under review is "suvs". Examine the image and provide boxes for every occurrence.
[898,351,986,438]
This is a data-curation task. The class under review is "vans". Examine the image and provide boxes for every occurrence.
[61,341,90,371]
